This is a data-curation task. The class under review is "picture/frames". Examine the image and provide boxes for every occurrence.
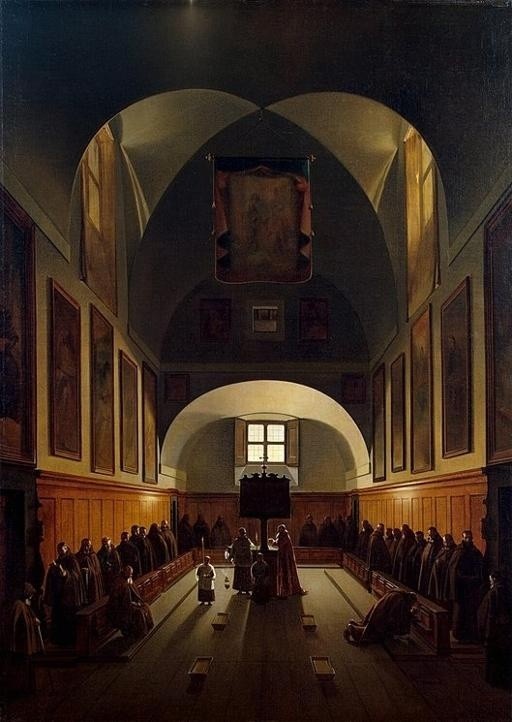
[164,374,190,402]
[118,347,140,475]
[141,359,158,484]
[480,192,512,471]
[389,352,406,474]
[244,298,287,342]
[340,375,367,404]
[88,299,117,479]
[200,300,232,342]
[374,361,386,481]
[439,274,473,461]
[407,302,437,474]
[299,296,329,342]
[47,273,84,464]
[0,178,40,470]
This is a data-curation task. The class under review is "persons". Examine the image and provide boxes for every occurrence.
[190,512,213,547]
[226,526,254,595]
[16,519,181,645]
[176,512,194,555]
[269,524,303,597]
[249,552,274,604]
[196,554,217,607]
[315,515,338,548]
[340,514,359,553]
[210,515,232,550]
[1,579,46,694]
[473,568,511,688]
[340,589,419,640]
[299,513,318,546]
[330,512,341,532]
[362,517,484,643]
